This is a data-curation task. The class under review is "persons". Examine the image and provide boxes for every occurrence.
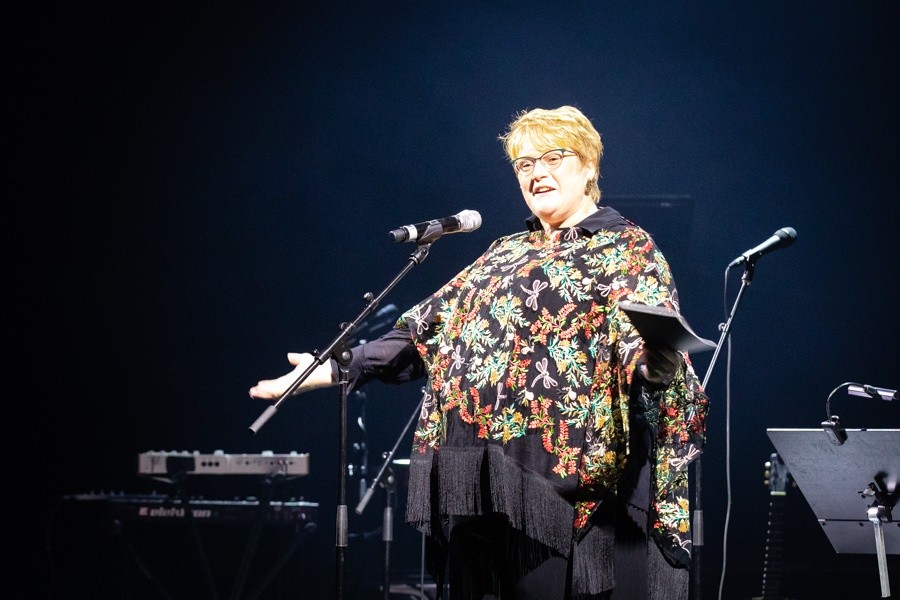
[248,105,714,600]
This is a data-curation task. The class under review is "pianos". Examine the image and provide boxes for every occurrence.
[60,490,322,599]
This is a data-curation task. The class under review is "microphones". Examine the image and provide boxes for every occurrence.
[729,227,798,266]
[388,210,481,244]
[347,304,397,346]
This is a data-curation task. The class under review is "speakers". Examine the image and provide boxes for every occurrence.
[59,494,321,600]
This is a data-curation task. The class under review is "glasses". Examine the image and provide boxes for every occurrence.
[510,148,579,174]
